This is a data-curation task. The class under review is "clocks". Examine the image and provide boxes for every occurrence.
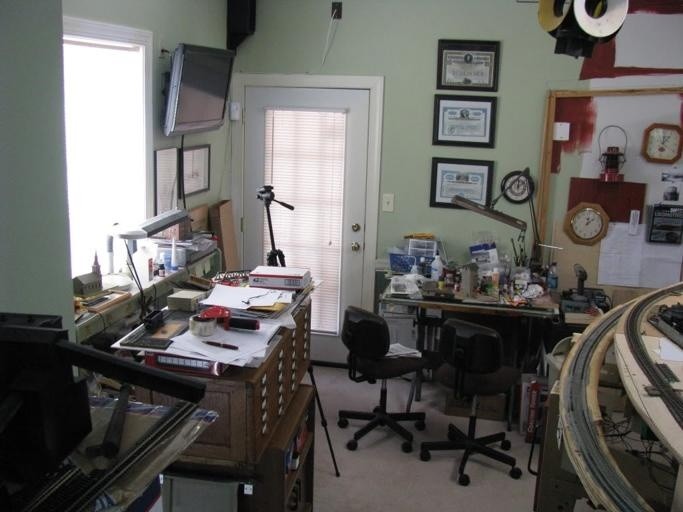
[562,202,610,245]
[641,123,683,164]
[501,170,535,204]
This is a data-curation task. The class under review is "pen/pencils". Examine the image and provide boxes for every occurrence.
[201,341,239,350]
[511,238,529,267]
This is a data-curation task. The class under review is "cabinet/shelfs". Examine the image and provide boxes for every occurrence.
[245,382,313,512]
[105,297,311,474]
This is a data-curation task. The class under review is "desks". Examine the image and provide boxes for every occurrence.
[380,279,559,401]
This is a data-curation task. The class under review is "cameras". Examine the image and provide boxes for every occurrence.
[256,186,274,200]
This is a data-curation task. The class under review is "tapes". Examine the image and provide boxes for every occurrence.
[189,314,217,337]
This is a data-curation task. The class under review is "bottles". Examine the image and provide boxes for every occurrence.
[158,264,165,277]
[547,262,558,292]
[431,256,515,295]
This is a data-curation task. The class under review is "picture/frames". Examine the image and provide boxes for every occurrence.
[177,143,211,199]
[432,93,497,149]
[436,39,500,91]
[430,157,494,210]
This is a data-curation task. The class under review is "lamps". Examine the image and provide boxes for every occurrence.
[537,0,630,59]
[451,167,543,274]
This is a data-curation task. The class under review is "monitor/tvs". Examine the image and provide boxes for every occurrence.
[164,43,235,136]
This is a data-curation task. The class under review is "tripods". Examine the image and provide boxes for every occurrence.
[268,250,340,476]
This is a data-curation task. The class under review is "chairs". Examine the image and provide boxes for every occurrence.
[421,318,522,486]
[337,305,426,452]
